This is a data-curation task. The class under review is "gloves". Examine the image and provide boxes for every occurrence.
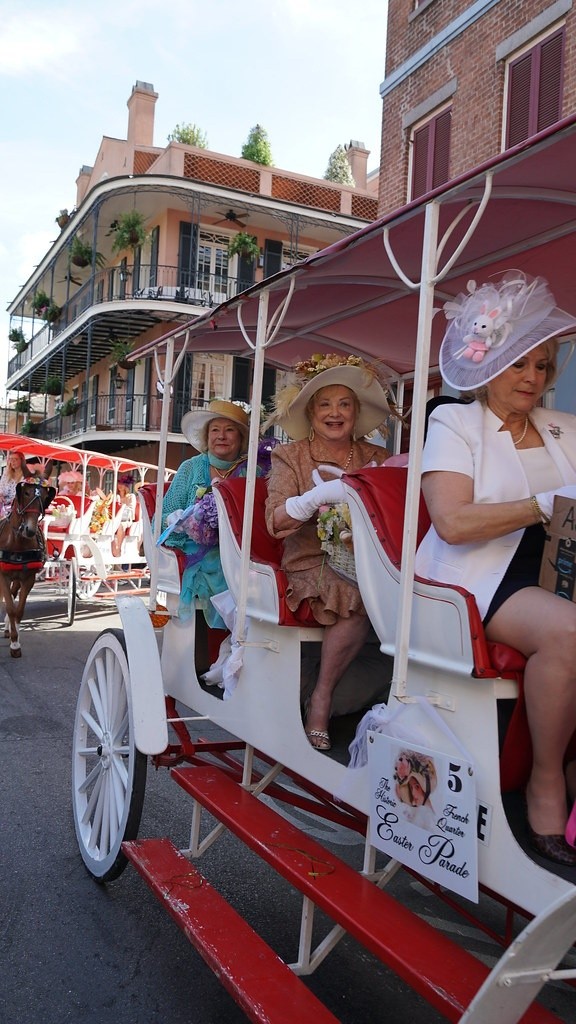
[285,479,348,522]
[534,485,576,519]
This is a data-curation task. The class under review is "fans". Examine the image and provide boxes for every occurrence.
[211,209,250,228]
[95,217,120,237]
[55,271,82,286]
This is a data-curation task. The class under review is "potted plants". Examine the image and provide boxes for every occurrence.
[59,396,79,415]
[68,235,107,271]
[14,374,62,412]
[109,210,147,257]
[109,338,137,372]
[21,419,40,435]
[230,230,260,258]
[10,290,60,352]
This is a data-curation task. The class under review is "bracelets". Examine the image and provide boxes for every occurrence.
[532,496,549,522]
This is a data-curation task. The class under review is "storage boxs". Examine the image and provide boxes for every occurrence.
[539,494,576,603]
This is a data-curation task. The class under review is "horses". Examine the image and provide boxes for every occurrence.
[0,472,56,658]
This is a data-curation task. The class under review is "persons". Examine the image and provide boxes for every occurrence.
[111,474,136,554]
[59,471,84,496]
[264,371,393,750]
[0,451,33,522]
[158,406,263,686]
[407,323,576,863]
[33,469,43,479]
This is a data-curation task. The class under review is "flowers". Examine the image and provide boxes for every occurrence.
[181,485,221,547]
[291,353,368,380]
[313,504,352,556]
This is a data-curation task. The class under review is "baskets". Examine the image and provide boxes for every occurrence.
[325,511,358,582]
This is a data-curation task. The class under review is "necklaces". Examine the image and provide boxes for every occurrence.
[215,467,225,478]
[514,417,528,445]
[343,444,353,470]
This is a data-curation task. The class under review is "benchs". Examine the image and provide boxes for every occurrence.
[38,493,143,560]
[338,465,525,845]
[210,478,371,737]
[137,479,225,688]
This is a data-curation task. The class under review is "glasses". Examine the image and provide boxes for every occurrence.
[9,458,20,461]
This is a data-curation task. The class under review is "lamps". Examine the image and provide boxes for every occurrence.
[115,373,128,389]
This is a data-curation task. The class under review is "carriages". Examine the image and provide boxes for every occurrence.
[0,431,176,660]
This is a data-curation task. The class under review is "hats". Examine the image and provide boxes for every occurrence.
[62,470,84,482]
[439,278,576,391]
[180,401,252,457]
[117,473,136,492]
[261,355,405,441]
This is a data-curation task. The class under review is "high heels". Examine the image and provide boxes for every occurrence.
[521,777,576,867]
[303,693,331,751]
